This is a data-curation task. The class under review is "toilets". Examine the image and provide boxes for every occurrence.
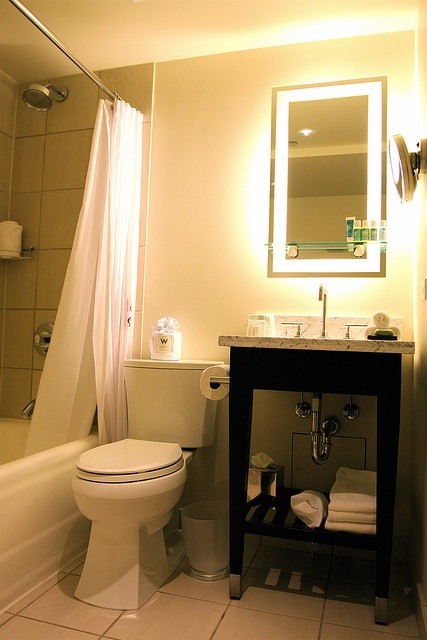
[72,358,225,610]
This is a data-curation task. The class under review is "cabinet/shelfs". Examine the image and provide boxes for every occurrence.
[226,346,404,625]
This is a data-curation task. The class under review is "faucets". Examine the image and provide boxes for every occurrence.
[20,398,36,418]
[318,284,327,336]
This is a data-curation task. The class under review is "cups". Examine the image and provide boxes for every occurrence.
[245,314,274,337]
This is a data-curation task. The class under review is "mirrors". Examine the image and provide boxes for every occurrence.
[265,73,389,278]
[388,133,417,204]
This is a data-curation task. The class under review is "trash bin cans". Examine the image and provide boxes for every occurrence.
[181,502,230,584]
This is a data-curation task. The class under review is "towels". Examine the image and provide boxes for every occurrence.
[324,509,378,524]
[372,311,390,328]
[0,220,24,260]
[363,326,377,339]
[327,465,378,513]
[388,326,402,339]
[323,522,379,536]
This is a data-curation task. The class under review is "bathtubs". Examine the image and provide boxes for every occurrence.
[0,419,102,615]
